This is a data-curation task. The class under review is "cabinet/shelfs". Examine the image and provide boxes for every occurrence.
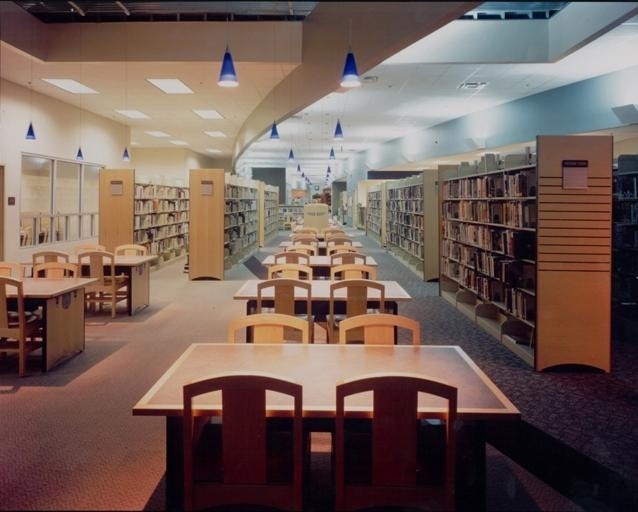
[279,201,328,228]
[99,169,279,281]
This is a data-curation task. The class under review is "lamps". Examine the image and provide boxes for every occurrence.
[324,166,332,187]
[270,9,280,140]
[329,142,335,159]
[288,118,314,187]
[217,10,241,88]
[340,26,363,88]
[334,92,345,139]
[24,14,131,163]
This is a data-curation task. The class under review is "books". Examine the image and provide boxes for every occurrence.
[611,174,638,321]
[366,191,382,237]
[263,190,277,240]
[439,168,537,321]
[503,331,530,345]
[133,182,189,258]
[224,186,257,258]
[278,201,306,229]
[385,184,424,259]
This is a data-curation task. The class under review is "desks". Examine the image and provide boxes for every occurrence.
[0,277,100,372]
[132,343,521,511]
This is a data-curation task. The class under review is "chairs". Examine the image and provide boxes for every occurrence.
[336,377,457,512]
[0,243,150,319]
[228,228,420,344]
[0,276,39,377]
[183,376,312,512]
